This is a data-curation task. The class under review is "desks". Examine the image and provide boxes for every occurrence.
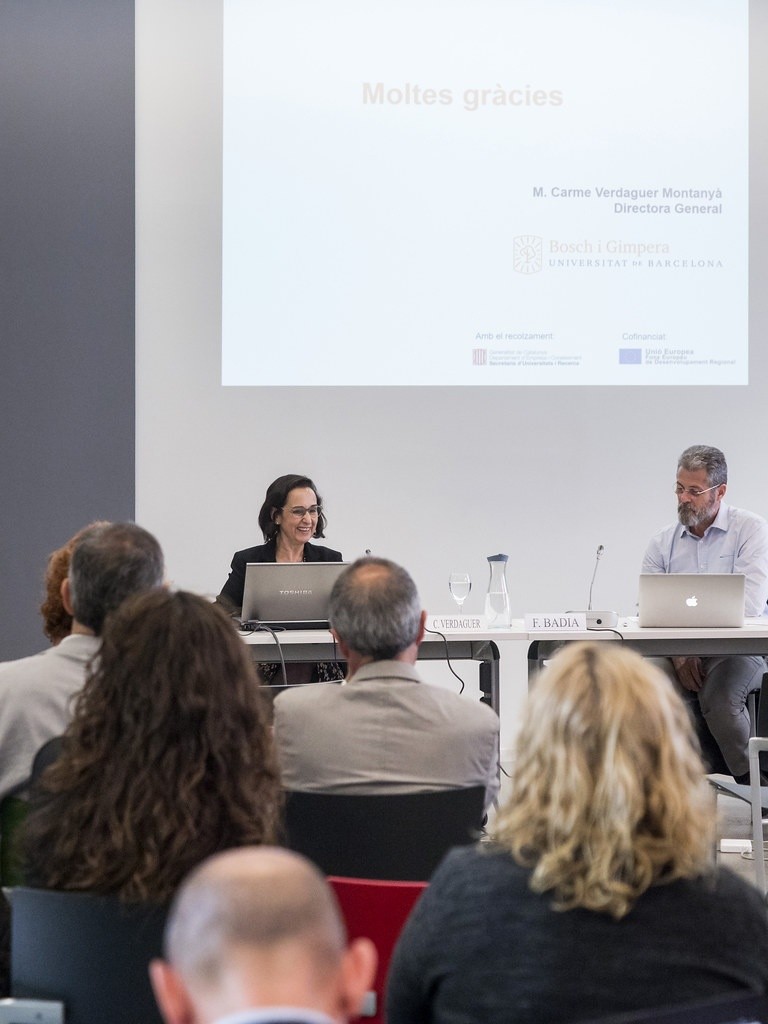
[243,632,502,734]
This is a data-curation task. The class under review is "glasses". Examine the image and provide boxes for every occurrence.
[672,482,721,498]
[281,505,324,519]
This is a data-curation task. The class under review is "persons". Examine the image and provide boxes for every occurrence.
[0,521,164,794]
[271,558,503,824]
[149,847,379,1023]
[383,643,768,1024]
[0,589,275,910]
[641,446,768,817]
[212,474,344,685]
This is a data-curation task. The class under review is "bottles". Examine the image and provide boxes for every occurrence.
[484,554,511,628]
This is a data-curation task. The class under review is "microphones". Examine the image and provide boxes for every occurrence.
[565,545,619,628]
[366,548,371,557]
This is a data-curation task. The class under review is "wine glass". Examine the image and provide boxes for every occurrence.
[449,573,471,621]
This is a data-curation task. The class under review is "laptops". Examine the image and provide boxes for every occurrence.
[231,561,353,630]
[639,573,746,627]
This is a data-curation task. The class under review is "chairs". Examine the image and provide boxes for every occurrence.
[698,735,768,899]
[527,630,768,791]
[280,783,483,886]
[321,877,438,1024]
[6,885,179,1024]
